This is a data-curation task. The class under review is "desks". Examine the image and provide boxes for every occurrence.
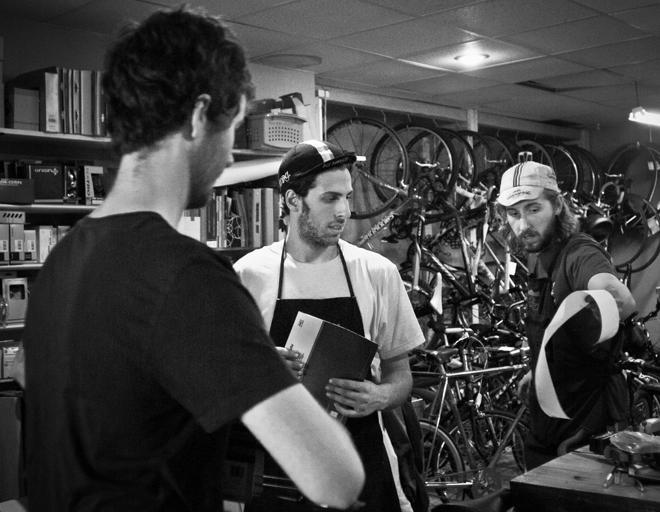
[509,436,658,511]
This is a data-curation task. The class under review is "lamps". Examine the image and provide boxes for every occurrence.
[628,79,660,129]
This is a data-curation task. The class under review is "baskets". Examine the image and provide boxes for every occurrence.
[246,113,307,152]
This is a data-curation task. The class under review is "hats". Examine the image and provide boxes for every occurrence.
[278,139,356,193]
[497,161,561,207]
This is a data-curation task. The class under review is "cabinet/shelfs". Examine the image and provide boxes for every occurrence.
[1,127,287,383]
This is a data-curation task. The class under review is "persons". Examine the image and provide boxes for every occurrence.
[21,3,366,511]
[496,160,639,475]
[229,138,427,512]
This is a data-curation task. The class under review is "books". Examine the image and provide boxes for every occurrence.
[284,311,379,425]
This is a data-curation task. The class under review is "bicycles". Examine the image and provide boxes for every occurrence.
[325,116,660,511]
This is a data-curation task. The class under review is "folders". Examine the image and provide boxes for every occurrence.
[245,188,262,248]
[262,188,274,245]
